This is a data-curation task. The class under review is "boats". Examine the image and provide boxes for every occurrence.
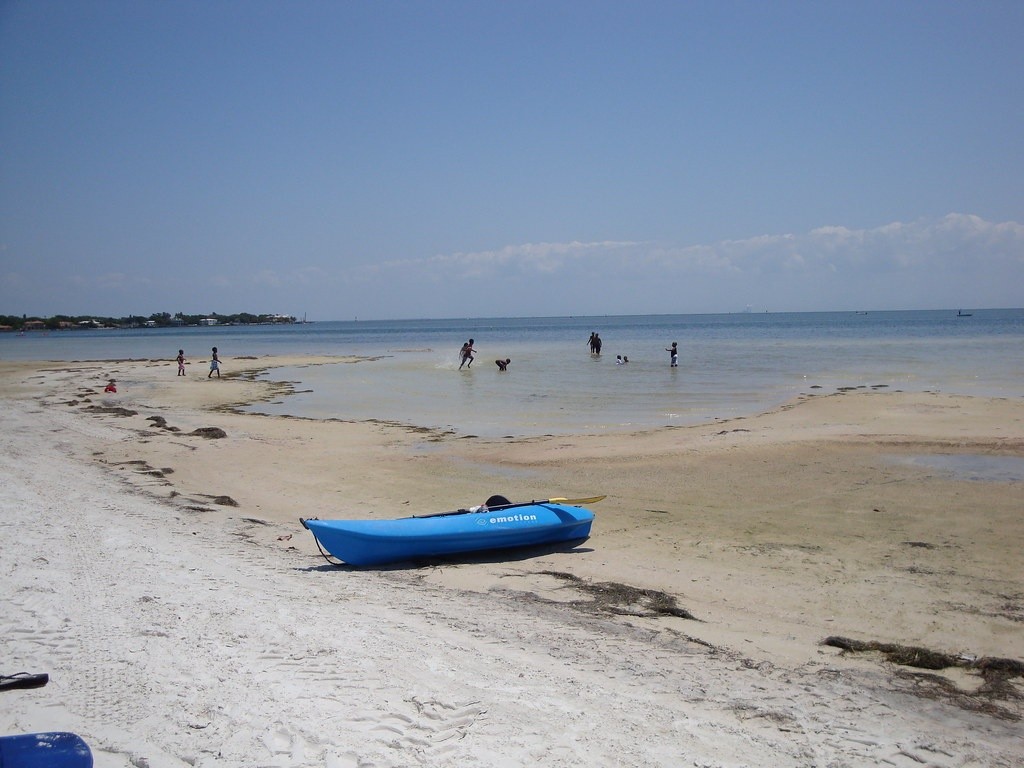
[299,494,595,568]
[956,313,972,317]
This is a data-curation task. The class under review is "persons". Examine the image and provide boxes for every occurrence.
[587,332,602,354]
[105,379,117,392]
[495,359,511,371]
[208,347,222,378]
[616,355,629,364]
[458,339,477,370]
[665,341,678,367]
[177,349,187,376]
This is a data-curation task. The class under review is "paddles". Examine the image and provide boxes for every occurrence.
[398,492,612,523]
